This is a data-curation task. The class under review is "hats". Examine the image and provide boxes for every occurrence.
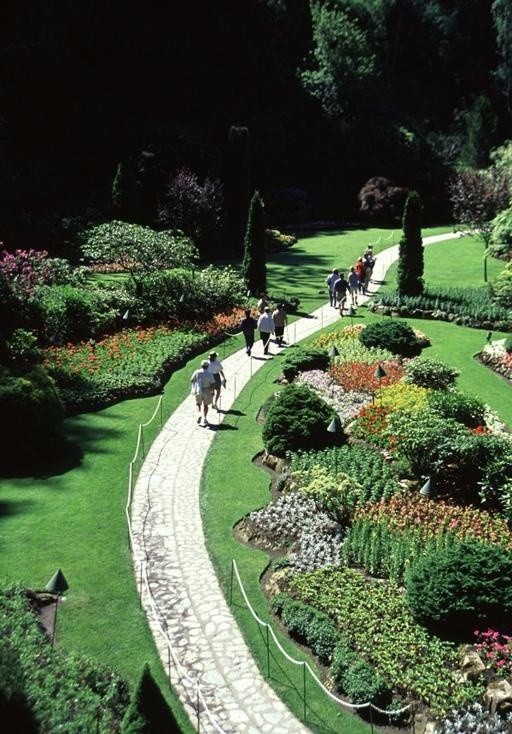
[201,351,219,367]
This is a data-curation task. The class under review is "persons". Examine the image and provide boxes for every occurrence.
[346,243,375,305]
[203,352,227,413]
[325,269,341,308]
[189,359,217,428]
[239,309,257,356]
[332,272,352,317]
[273,303,289,345]
[255,305,275,354]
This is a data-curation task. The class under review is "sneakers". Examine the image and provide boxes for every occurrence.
[197,405,218,424]
